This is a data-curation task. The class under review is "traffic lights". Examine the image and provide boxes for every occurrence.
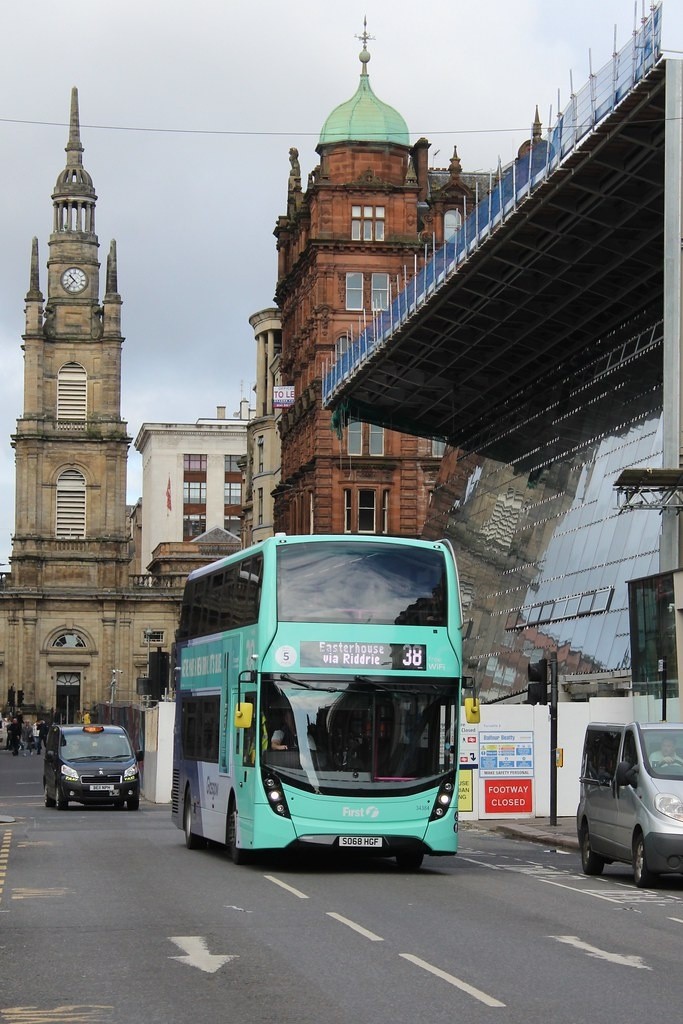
[528,658,547,706]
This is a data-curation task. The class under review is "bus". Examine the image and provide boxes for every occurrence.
[172,533,480,870]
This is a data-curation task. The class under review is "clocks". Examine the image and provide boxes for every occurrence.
[60,267,89,295]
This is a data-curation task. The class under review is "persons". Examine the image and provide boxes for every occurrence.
[649,738,683,767]
[84,712,91,724]
[271,710,317,751]
[0,711,49,755]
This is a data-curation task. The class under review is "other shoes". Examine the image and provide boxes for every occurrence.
[22,754,27,757]
[37,752,41,755]
[30,752,33,755]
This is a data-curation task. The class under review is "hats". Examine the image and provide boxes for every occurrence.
[36,717,44,722]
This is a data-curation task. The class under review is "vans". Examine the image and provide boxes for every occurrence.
[577,721,683,888]
[43,723,144,810]
